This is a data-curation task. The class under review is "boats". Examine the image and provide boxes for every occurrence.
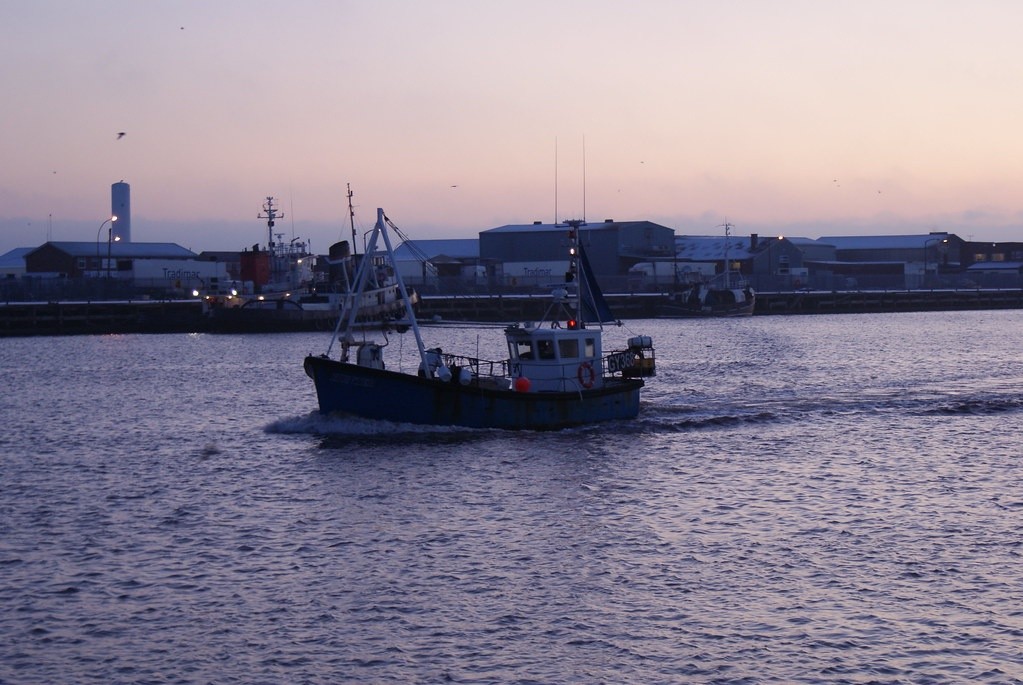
[202,179,418,330]
[303,201,657,426]
[672,220,755,320]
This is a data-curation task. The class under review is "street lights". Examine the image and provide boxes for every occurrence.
[766,232,784,294]
[95,213,118,308]
[924,235,949,289]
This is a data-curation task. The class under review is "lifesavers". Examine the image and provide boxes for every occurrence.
[579,363,594,388]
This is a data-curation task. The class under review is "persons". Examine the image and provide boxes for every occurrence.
[418,346,442,377]
